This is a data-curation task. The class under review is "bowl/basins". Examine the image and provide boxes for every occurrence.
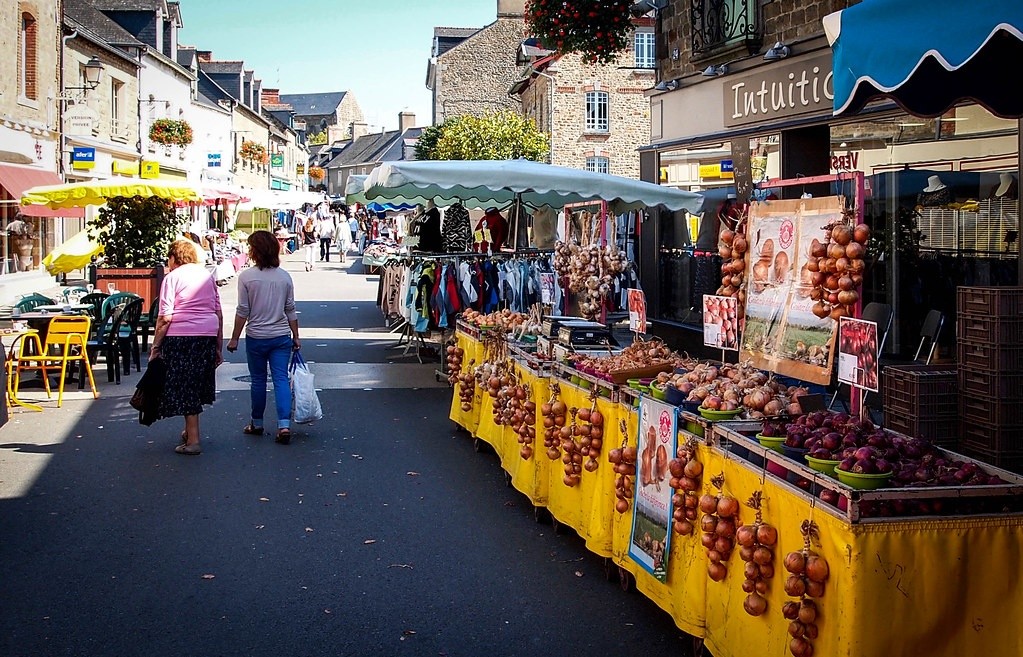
[638,385,651,394]
[697,405,741,421]
[626,378,640,389]
[804,455,843,478]
[650,379,667,400]
[756,433,787,455]
[833,466,893,490]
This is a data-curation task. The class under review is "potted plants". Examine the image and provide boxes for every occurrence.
[89,267,175,314]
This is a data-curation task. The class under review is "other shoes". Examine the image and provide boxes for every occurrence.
[181,429,188,442]
[175,443,202,456]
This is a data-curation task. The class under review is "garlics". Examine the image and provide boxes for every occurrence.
[557,240,630,317]
[506,320,542,343]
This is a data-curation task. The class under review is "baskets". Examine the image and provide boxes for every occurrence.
[609,361,673,384]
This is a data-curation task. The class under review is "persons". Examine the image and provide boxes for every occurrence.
[148,238,223,455]
[313,208,399,263]
[986,171,1019,286]
[227,231,301,446]
[914,175,956,360]
[6,211,35,241]
[301,217,318,270]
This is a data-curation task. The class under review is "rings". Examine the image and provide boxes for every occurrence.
[297,347,299,348]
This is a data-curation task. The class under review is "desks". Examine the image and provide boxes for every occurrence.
[0,312,95,390]
[33,306,96,376]
[448,316,1023,657]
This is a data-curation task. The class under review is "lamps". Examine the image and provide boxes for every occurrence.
[702,65,727,76]
[64,54,105,110]
[763,42,790,60]
[655,80,679,90]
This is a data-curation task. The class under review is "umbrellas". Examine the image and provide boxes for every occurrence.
[41,215,115,276]
[343,155,705,258]
[170,183,251,232]
[20,173,205,210]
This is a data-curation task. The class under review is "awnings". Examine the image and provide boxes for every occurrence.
[0,164,86,218]
[823,0,1023,119]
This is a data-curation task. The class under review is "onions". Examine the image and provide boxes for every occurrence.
[841,320,877,388]
[808,222,869,321]
[446,344,829,657]
[566,340,983,487]
[705,297,737,347]
[715,229,748,321]
[463,308,527,327]
[753,239,814,296]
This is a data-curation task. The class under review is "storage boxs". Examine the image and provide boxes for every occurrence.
[883,282,1023,476]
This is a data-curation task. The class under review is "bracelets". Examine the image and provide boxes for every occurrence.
[152,345,161,349]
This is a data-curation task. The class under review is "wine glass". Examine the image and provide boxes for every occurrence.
[108,283,115,295]
[86,284,94,295]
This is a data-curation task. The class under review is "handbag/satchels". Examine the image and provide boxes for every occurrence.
[289,350,323,423]
[128,355,168,424]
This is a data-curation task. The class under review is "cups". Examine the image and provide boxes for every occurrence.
[12,324,23,331]
[13,308,20,316]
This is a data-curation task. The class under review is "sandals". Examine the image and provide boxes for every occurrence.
[243,424,264,436]
[276,428,291,442]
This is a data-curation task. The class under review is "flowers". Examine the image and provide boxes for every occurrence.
[81,194,180,272]
[150,118,324,178]
[523,0,640,65]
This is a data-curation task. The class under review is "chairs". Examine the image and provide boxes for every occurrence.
[829,303,945,420]
[4,285,160,408]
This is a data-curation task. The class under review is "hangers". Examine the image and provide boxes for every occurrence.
[389,250,554,268]
